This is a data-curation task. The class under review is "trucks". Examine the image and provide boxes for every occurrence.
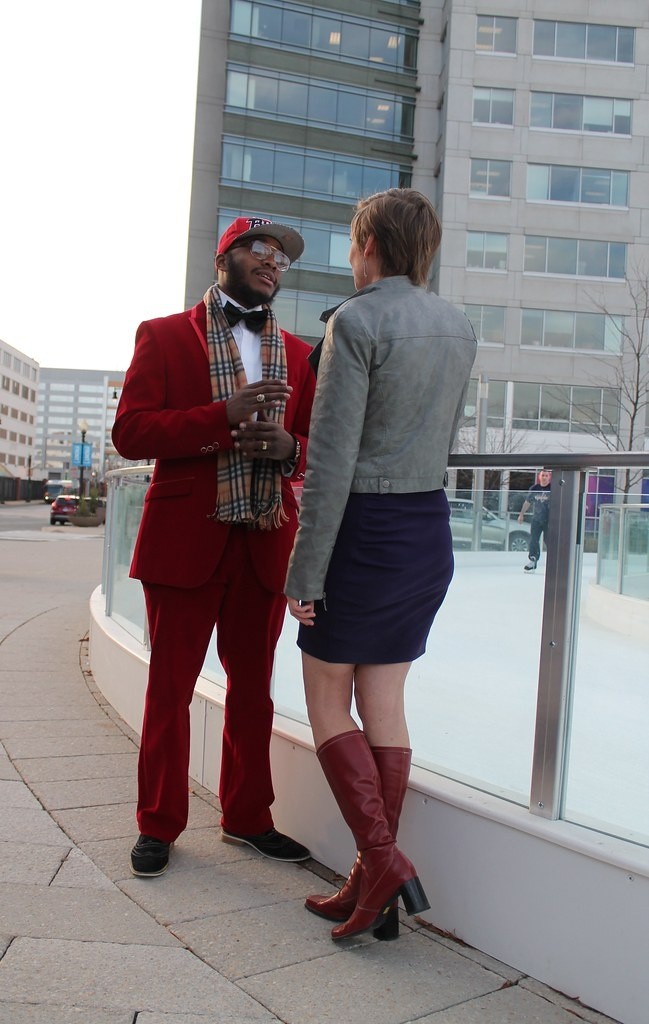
[43,479,73,503]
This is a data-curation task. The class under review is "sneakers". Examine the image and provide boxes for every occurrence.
[524,555,537,574]
[221,827,311,861]
[129,833,174,876]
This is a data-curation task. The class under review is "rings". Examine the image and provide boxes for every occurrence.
[262,441,267,450]
[256,395,265,403]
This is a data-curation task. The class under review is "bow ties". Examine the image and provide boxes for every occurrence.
[222,300,268,333]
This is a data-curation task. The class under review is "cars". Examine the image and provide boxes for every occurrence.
[50,495,81,526]
[447,497,532,552]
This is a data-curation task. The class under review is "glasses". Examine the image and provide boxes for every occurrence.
[224,240,290,272]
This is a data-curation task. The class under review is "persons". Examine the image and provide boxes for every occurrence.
[517,469,552,569]
[113,216,317,878]
[282,189,476,944]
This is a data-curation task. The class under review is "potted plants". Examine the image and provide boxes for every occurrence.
[67,480,106,527]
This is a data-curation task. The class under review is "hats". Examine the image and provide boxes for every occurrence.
[216,217,305,264]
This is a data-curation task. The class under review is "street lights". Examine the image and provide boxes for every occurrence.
[77,417,89,499]
[98,375,118,478]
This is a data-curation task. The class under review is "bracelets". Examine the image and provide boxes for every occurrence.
[288,437,300,464]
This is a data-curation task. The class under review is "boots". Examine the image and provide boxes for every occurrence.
[316,729,431,940]
[305,745,412,940]
